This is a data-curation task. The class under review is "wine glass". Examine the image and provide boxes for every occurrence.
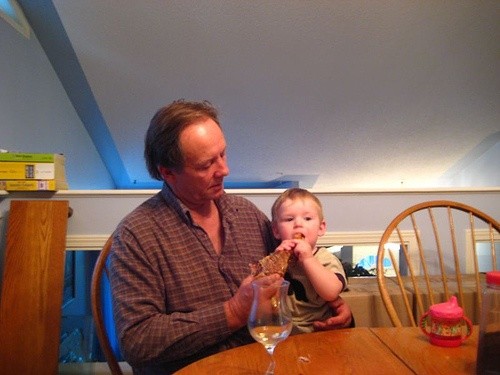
[246,279,294,375]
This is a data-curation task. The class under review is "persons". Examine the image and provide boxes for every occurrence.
[269,188,347,335]
[110,98,356,375]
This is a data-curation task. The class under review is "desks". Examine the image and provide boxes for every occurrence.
[338,272,500,326]
[173,322,499,375]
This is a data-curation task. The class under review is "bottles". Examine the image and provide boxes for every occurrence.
[420,296,473,348]
[476,270,500,375]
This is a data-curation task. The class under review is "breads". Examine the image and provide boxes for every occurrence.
[249,233,304,280]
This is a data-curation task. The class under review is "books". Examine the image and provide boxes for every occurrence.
[0,149,69,192]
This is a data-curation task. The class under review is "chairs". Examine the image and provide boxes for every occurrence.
[377,201,500,327]
